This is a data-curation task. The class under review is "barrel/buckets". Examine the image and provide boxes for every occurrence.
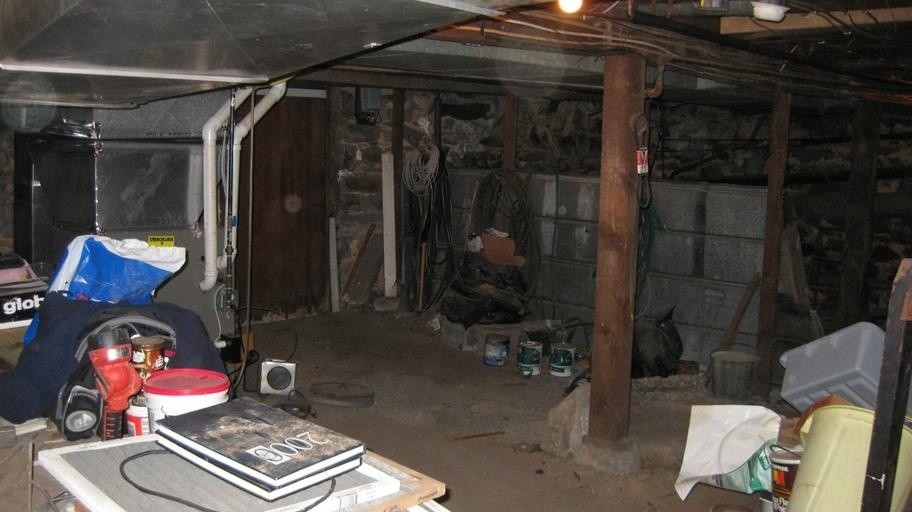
[709,342,763,398]
[784,404,912,512]
[768,445,803,512]
[548,343,575,378]
[518,341,541,376]
[485,333,509,366]
[142,368,232,434]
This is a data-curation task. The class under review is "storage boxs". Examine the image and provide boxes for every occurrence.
[778,320,912,427]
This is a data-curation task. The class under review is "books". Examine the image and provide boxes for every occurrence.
[153,394,363,503]
